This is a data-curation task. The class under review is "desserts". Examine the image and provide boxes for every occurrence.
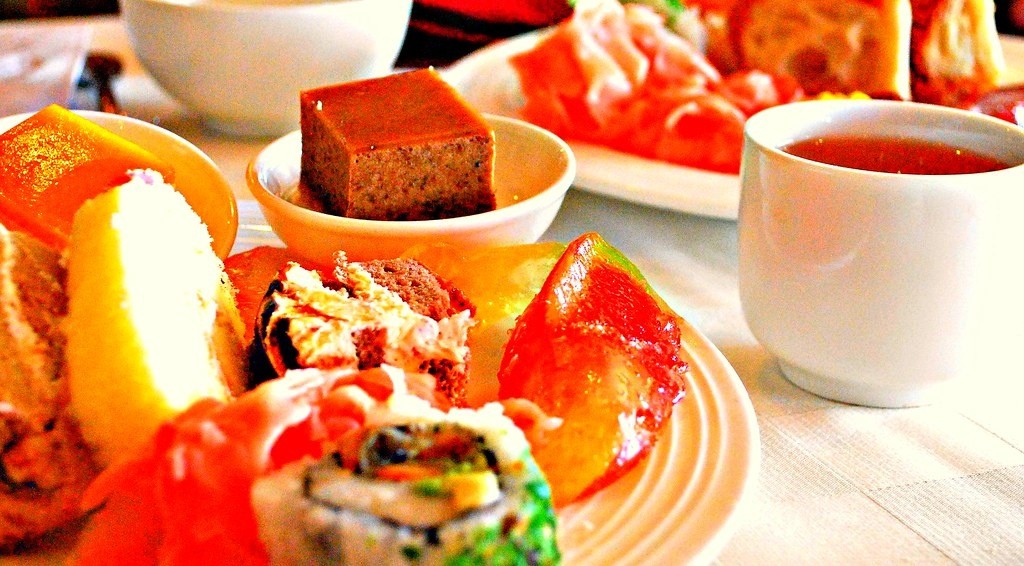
[298,66,496,222]
[0,104,175,247]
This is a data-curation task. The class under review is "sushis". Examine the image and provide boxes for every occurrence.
[297,398,563,566]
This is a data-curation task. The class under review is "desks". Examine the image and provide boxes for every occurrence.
[0,16,1024,566]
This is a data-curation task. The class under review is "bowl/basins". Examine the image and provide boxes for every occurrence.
[0,110,236,262]
[246,112,576,266]
[122,1,412,137]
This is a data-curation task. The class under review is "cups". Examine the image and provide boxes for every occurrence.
[738,100,1023,407]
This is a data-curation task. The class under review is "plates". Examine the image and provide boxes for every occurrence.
[557,328,764,566]
[440,23,1023,218]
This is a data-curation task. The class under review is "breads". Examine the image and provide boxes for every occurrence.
[708,0,1004,102]
[0,169,479,554]
